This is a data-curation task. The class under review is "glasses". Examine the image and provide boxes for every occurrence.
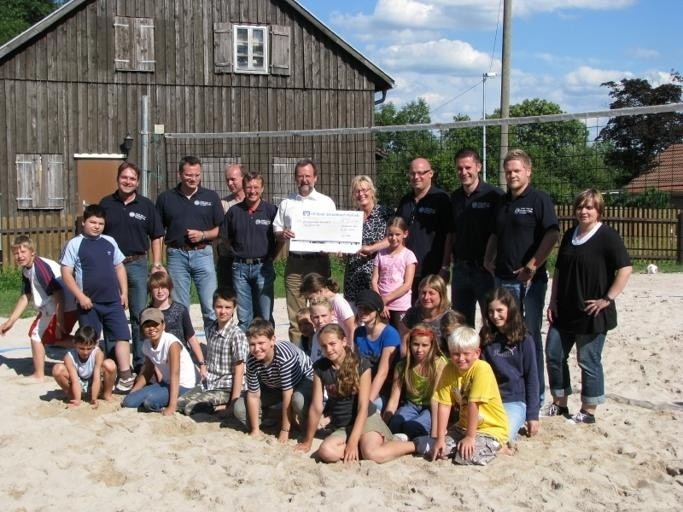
[355,188,371,193]
[410,170,430,175]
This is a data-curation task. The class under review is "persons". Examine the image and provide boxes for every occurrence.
[1,147,633,472]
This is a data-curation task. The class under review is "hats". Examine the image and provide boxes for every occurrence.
[141,307,164,326]
[356,290,383,312]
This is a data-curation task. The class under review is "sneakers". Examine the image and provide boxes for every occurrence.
[566,409,595,424]
[414,436,436,455]
[539,403,568,417]
[116,373,137,391]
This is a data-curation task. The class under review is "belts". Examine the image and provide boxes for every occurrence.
[235,258,265,264]
[174,243,208,250]
[122,252,148,264]
[289,252,322,259]
